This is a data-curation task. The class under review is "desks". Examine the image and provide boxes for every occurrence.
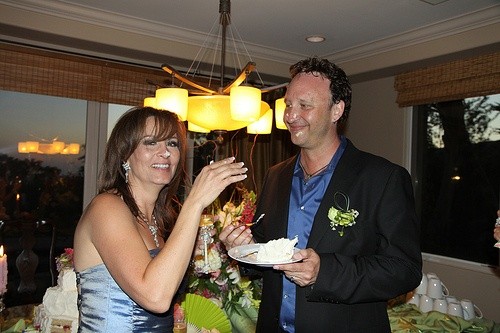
[0,304,41,333]
[224,290,500,333]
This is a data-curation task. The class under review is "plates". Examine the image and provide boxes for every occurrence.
[228,244,302,266]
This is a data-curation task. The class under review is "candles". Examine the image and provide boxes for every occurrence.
[0,244,8,295]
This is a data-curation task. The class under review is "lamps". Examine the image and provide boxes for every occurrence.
[18,134,80,154]
[144,0,288,134]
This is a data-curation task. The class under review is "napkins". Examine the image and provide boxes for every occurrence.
[3,319,25,333]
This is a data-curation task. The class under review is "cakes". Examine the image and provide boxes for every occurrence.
[257,236,298,262]
[42,267,79,320]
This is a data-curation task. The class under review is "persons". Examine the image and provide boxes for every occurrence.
[73,107,248,333]
[218,57,422,333]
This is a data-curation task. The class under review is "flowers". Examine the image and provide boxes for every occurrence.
[328,191,359,236]
[55,248,74,272]
[185,184,263,319]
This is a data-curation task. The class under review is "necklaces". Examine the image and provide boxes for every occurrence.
[137,210,161,247]
[299,161,328,185]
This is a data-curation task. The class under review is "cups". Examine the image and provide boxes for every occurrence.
[445,295,459,304]
[414,273,426,295]
[420,295,434,313]
[449,303,462,318]
[426,278,449,299]
[461,299,483,320]
[406,294,421,308]
[426,272,438,280]
[433,299,447,315]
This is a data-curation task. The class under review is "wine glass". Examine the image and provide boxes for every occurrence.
[197,202,217,273]
